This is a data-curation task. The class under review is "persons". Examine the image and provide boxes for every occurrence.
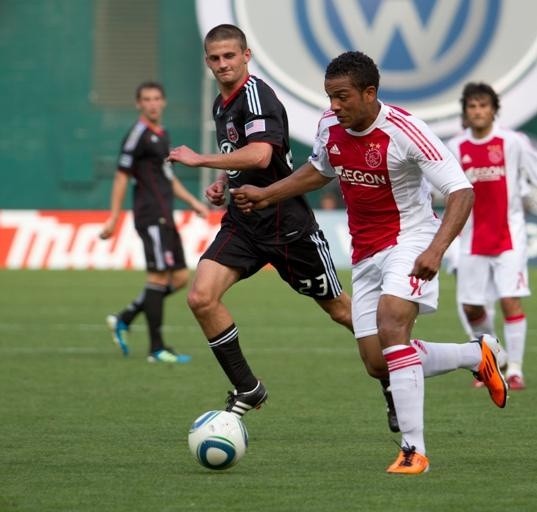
[189,25,401,432]
[432,82,537,390]
[229,53,508,475]
[100,82,208,363]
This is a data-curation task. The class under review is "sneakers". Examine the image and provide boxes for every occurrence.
[504,367,525,391]
[379,377,402,433]
[105,313,133,359]
[470,374,486,388]
[467,332,511,410]
[386,447,429,475]
[225,380,269,417]
[146,347,191,365]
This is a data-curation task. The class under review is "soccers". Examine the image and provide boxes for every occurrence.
[188,410,248,469]
